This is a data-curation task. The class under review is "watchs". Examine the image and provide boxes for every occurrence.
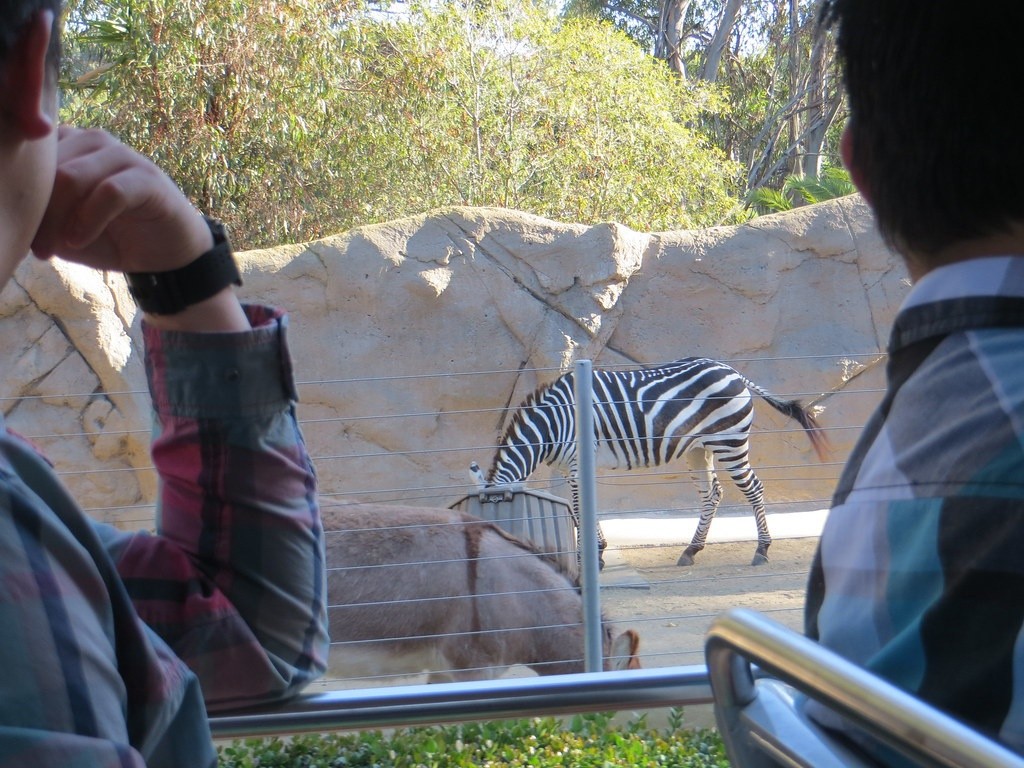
[124,217,242,313]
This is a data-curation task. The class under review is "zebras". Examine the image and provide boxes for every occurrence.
[468,357,832,568]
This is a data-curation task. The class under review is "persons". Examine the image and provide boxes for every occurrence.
[799,0,1024,768]
[0,1,328,767]
